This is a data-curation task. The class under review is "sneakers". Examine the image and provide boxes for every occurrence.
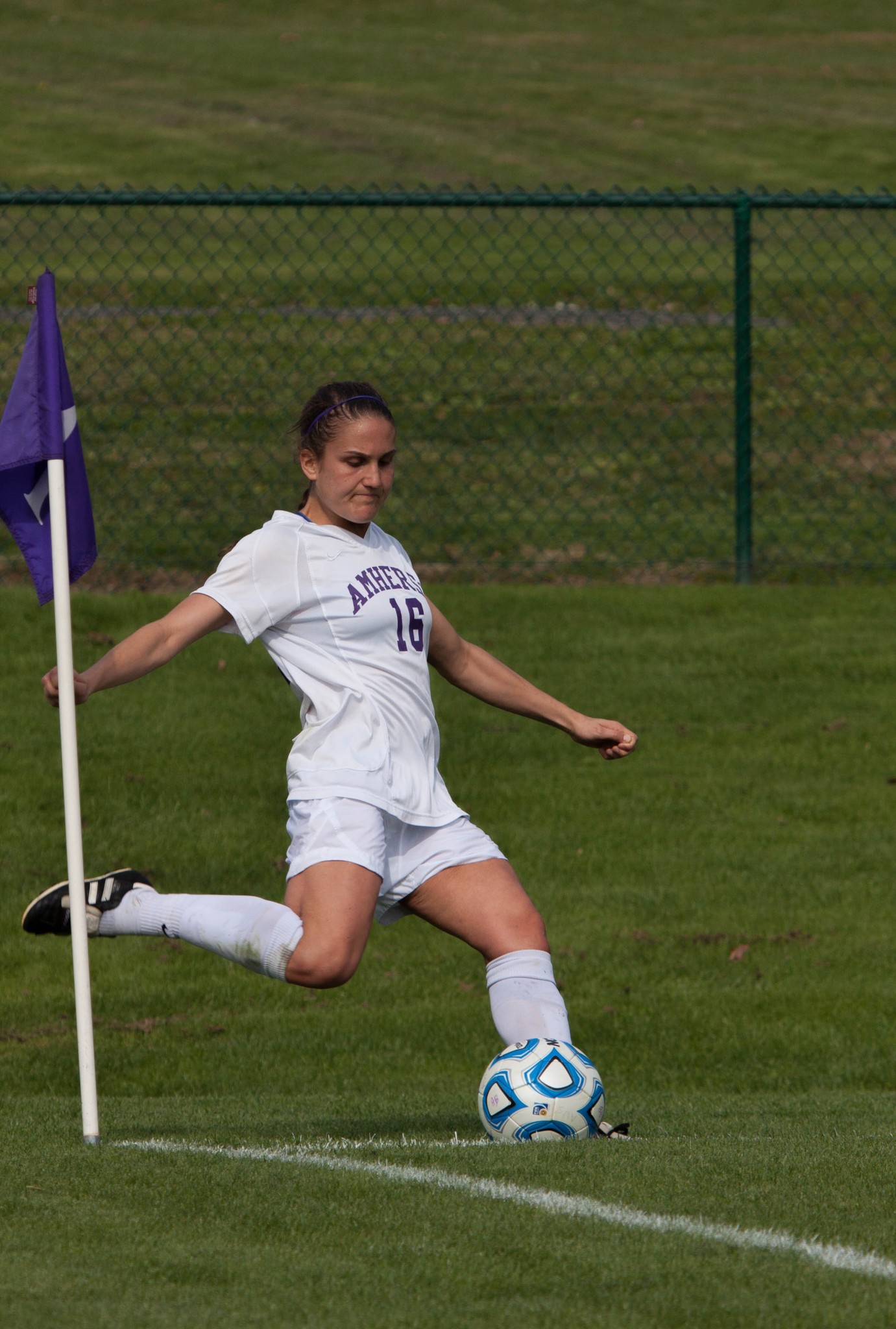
[22,866,154,939]
[593,1114,632,1141]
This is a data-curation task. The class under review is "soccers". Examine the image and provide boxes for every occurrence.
[469,1033,604,1147]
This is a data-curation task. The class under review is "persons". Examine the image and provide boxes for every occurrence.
[0,275,97,604]
[20,384,640,1140]
